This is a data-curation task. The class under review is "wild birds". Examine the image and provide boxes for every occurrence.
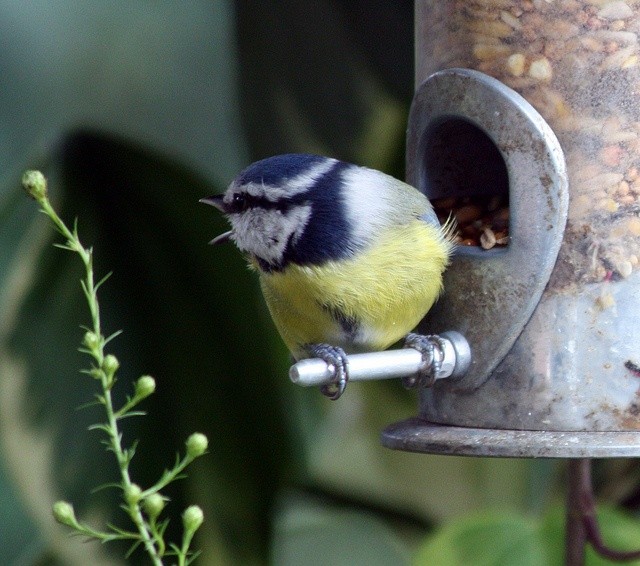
[197,151,462,401]
